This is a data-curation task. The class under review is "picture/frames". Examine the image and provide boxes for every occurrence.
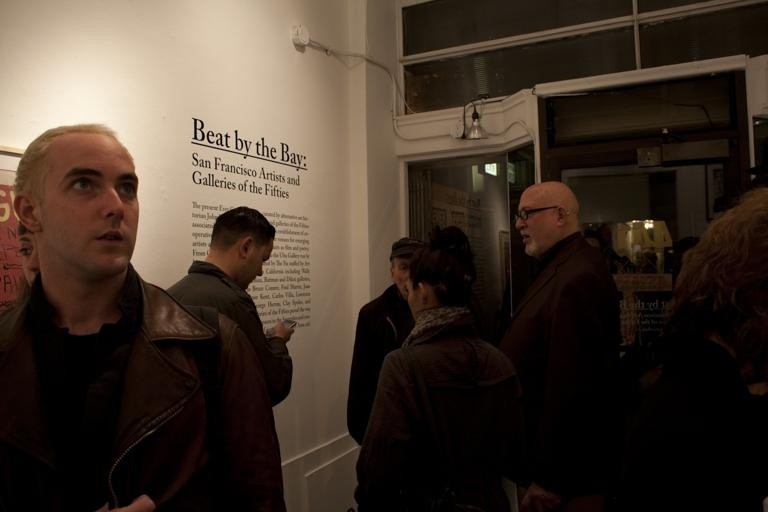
[704,164,724,221]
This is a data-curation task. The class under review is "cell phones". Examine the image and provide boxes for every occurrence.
[265,319,297,336]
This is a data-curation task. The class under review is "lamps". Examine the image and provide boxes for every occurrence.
[461,94,487,140]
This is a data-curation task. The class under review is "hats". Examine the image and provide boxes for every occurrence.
[390,237,421,256]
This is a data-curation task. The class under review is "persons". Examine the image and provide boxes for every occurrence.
[167,204,295,408]
[345,224,522,512]
[0,218,40,324]
[496,180,623,510]
[0,121,289,512]
[346,235,429,446]
[590,183,768,512]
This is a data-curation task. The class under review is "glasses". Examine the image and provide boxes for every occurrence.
[511,206,558,222]
